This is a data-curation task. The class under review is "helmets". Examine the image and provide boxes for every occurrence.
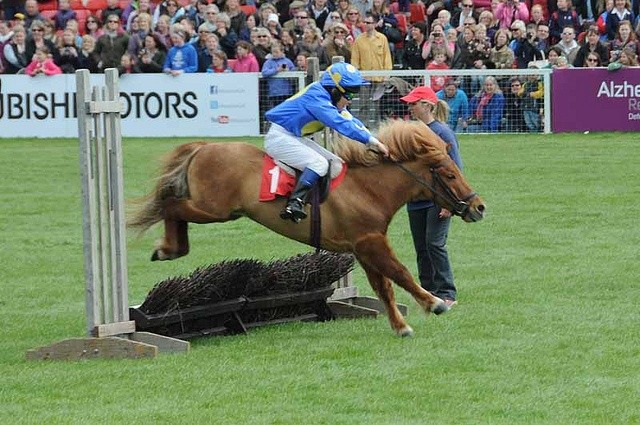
[321,61,372,88]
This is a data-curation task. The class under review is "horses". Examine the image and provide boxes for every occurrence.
[122,116,487,338]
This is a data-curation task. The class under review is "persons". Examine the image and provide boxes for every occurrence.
[321,9,352,48]
[515,24,543,69]
[25,45,62,76]
[283,1,315,31]
[431,10,454,30]
[251,28,276,72]
[434,80,469,131]
[597,0,615,42]
[225,0,246,35]
[478,11,497,47]
[581,51,604,67]
[605,0,634,37]
[349,13,394,127]
[505,19,527,52]
[290,11,317,40]
[5,25,38,74]
[527,4,549,44]
[573,25,607,67]
[159,0,185,23]
[319,23,352,69]
[634,13,640,38]
[197,34,227,72]
[543,46,561,68]
[293,24,331,70]
[491,0,529,29]
[163,30,200,76]
[518,66,544,132]
[197,4,219,33]
[154,0,168,22]
[188,0,208,32]
[50,0,77,28]
[464,46,497,69]
[0,21,12,73]
[425,48,450,70]
[552,57,574,69]
[86,15,105,39]
[52,28,79,73]
[206,50,234,73]
[555,26,580,64]
[76,35,99,72]
[450,0,481,28]
[156,14,175,48]
[421,23,454,59]
[181,18,212,72]
[333,0,360,21]
[366,0,398,55]
[405,22,429,70]
[532,21,552,49]
[24,20,63,66]
[280,29,309,64]
[468,76,504,133]
[489,30,514,67]
[129,13,167,61]
[607,46,640,70]
[457,17,475,44]
[126,0,155,35]
[547,0,578,40]
[504,77,525,129]
[446,29,458,56]
[212,12,239,59]
[23,0,47,29]
[411,0,445,26]
[231,40,261,72]
[10,13,35,65]
[305,0,333,31]
[261,41,297,107]
[604,20,640,65]
[263,62,371,221]
[292,52,311,71]
[137,34,168,73]
[65,20,79,33]
[341,7,366,42]
[468,23,491,55]
[101,0,124,24]
[522,0,550,22]
[457,27,478,60]
[400,86,461,310]
[258,3,282,39]
[120,55,139,74]
[239,13,261,42]
[92,14,134,74]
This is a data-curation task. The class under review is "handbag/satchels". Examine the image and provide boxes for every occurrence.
[384,23,402,43]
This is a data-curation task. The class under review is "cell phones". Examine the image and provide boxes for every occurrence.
[433,33,441,37]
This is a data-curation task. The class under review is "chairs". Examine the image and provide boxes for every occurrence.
[41,10,56,17]
[38,1,58,12]
[75,10,90,34]
[395,14,407,48]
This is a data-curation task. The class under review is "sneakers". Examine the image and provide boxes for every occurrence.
[444,297,455,306]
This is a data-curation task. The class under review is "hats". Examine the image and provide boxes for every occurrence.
[399,86,440,105]
[330,22,349,35]
[289,2,303,9]
[8,13,26,21]
[267,13,279,23]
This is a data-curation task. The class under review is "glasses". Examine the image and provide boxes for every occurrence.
[199,30,210,34]
[347,12,358,16]
[330,15,339,19]
[86,20,96,24]
[108,20,118,24]
[587,58,598,62]
[363,20,374,25]
[207,12,215,15]
[538,29,549,33]
[167,3,177,7]
[328,72,355,101]
[562,33,574,35]
[258,34,268,38]
[510,28,520,32]
[462,4,474,8]
[335,30,344,34]
[295,15,308,19]
[32,28,42,32]
[463,22,475,26]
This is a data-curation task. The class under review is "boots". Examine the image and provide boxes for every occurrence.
[279,168,322,219]
